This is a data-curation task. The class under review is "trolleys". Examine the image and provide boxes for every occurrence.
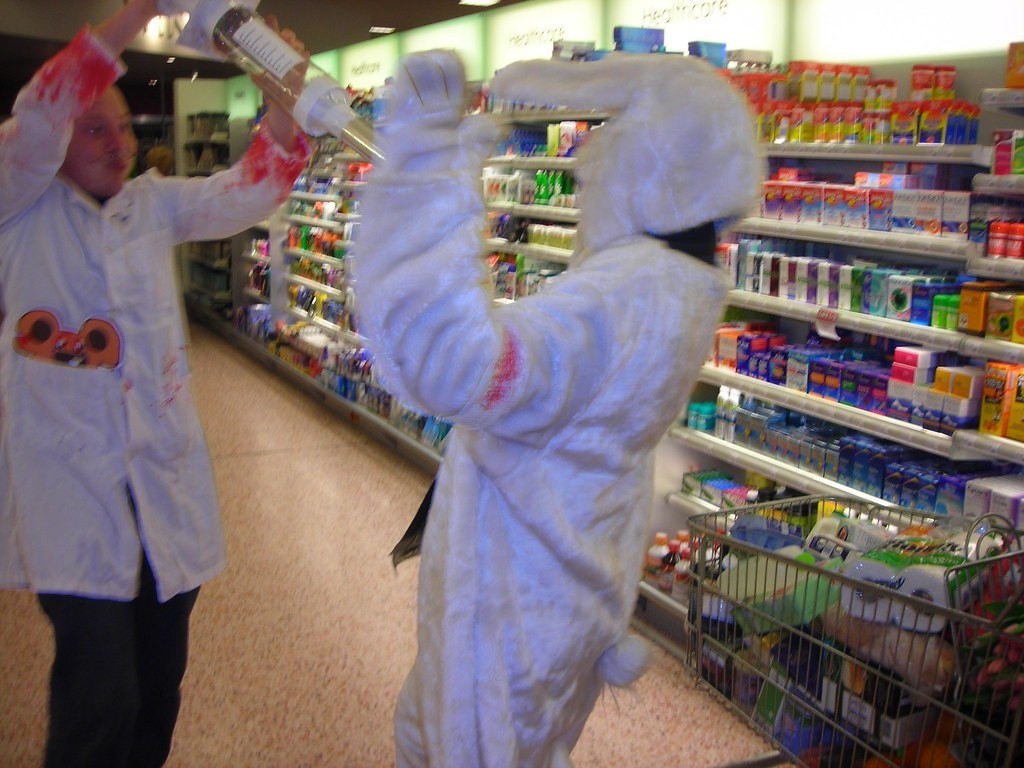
[687,490,1024,768]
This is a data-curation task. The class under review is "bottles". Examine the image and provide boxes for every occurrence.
[730,637,762,714]
[211,7,381,163]
[647,528,725,604]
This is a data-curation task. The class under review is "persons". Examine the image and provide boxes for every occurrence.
[0,1,319,768]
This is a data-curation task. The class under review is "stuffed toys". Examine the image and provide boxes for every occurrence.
[348,49,766,768]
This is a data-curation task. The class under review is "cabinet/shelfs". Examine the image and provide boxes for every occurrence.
[129,51,1024,660]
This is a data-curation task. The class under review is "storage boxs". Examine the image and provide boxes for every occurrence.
[247,27,1023,539]
[750,664,932,749]
